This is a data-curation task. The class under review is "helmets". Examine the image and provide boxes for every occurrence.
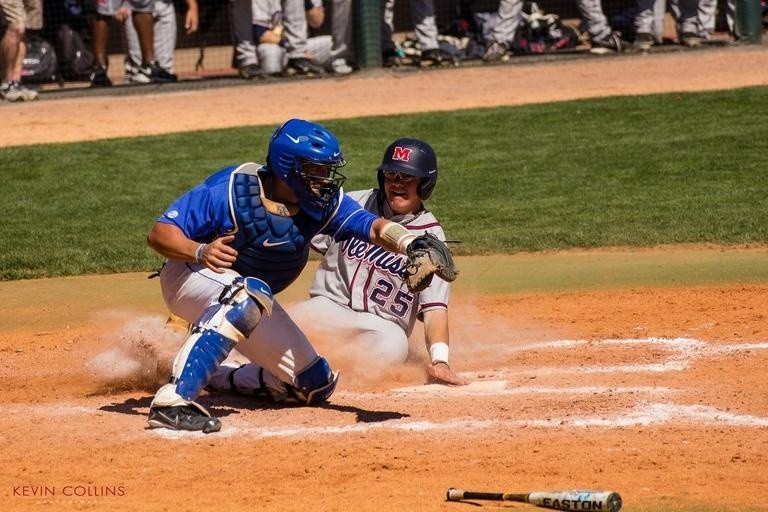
[267,118,347,220]
[376,138,437,200]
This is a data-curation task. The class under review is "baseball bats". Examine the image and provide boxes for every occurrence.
[447,487,623,512]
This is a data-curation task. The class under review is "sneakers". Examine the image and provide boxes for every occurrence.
[421,47,454,67]
[88,66,112,87]
[139,60,177,82]
[1,80,38,102]
[286,58,324,78]
[148,406,221,433]
[482,42,508,62]
[589,31,653,55]
[237,65,269,81]
[681,32,702,47]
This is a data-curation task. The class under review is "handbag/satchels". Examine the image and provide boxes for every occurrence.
[512,2,577,53]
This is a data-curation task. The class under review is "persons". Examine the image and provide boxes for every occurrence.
[202,135,471,404]
[145,117,459,434]
[1,1,767,102]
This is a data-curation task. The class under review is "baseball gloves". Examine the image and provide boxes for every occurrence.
[405,230,459,294]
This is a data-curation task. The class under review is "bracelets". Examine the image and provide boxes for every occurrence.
[429,341,449,367]
[195,243,208,269]
[378,219,410,255]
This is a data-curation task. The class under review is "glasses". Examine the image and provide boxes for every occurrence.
[383,170,415,181]
